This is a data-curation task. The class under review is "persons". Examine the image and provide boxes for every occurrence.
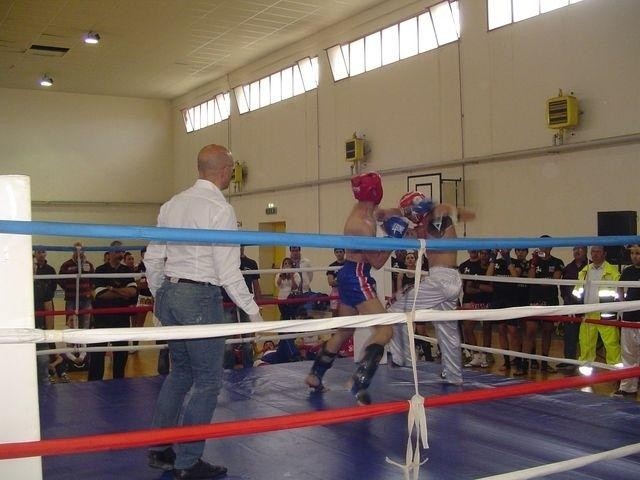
[386,235,640,398]
[141,144,264,480]
[385,191,464,386]
[220,244,347,369]
[305,171,435,405]
[33,241,170,384]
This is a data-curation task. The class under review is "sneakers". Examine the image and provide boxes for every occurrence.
[176,459,228,479]
[612,390,638,397]
[148,448,175,472]
[464,349,577,375]
[48,373,72,384]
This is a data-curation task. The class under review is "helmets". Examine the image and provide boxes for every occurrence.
[351,172,383,204]
[399,191,432,223]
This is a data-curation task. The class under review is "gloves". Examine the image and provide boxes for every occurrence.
[382,215,409,239]
[153,301,164,328]
[250,312,266,344]
[428,215,454,238]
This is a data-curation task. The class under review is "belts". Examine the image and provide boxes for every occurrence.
[165,274,215,286]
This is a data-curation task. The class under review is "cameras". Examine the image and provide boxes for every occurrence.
[537,252,545,257]
